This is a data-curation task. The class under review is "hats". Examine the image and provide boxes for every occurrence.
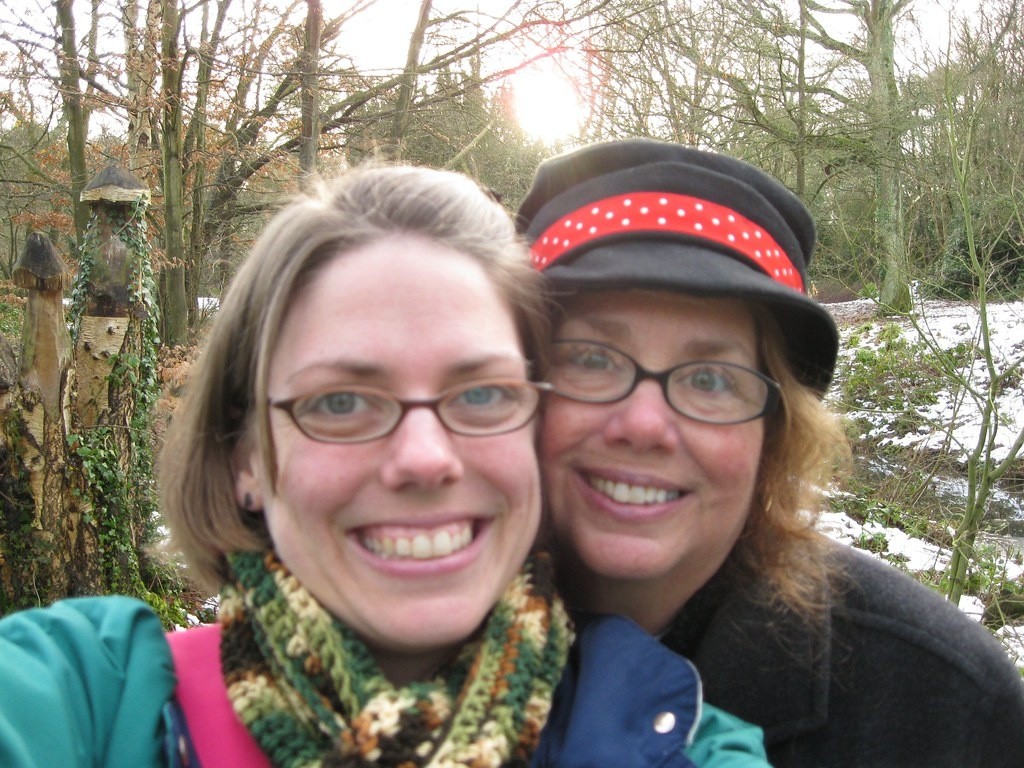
[516,137,840,401]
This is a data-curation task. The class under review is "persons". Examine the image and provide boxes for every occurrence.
[513,136,1024,768]
[0,162,772,768]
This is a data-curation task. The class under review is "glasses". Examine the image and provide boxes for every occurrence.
[535,338,785,425]
[269,373,554,446]
